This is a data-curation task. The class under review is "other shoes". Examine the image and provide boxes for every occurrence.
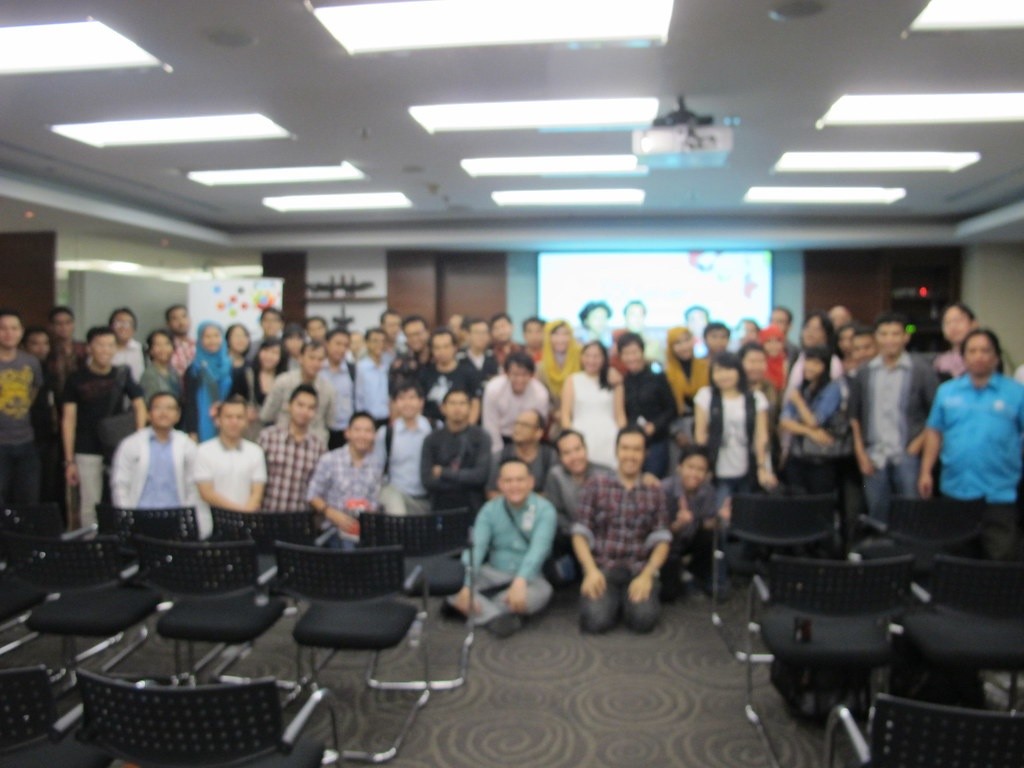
[489,613,522,637]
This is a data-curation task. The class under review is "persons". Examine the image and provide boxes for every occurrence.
[307,411,382,545]
[543,429,617,614]
[917,327,1024,561]
[570,424,674,634]
[0,300,880,536]
[659,444,736,605]
[933,302,982,379]
[848,310,935,526]
[445,458,558,638]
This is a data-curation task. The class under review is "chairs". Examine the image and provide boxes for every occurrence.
[712,491,1024,768]
[0,503,474,768]
